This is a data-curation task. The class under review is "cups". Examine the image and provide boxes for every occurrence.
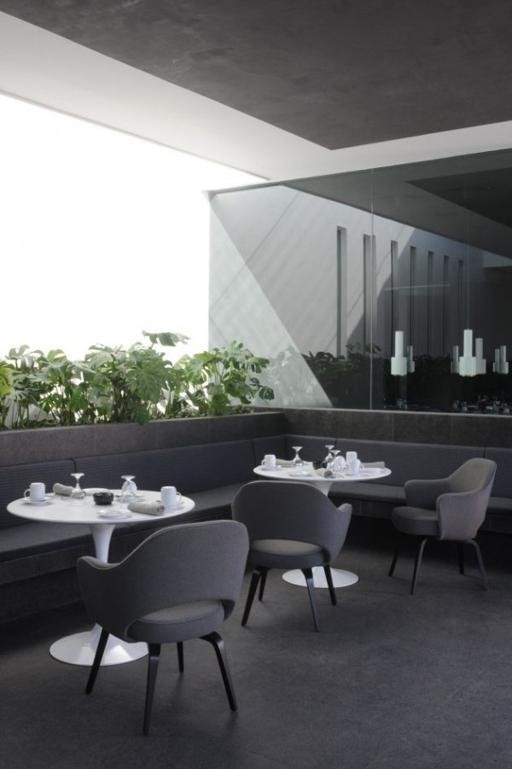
[350,459,364,473]
[23,482,46,501]
[160,485,182,508]
[261,454,276,468]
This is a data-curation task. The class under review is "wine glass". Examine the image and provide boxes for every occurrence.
[325,444,334,464]
[292,446,303,467]
[121,476,139,503]
[70,472,86,504]
[330,450,341,472]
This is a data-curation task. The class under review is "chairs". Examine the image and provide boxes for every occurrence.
[226,479,355,635]
[387,455,501,595]
[71,514,257,741]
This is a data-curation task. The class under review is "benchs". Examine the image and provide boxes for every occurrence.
[287,432,510,544]
[0,433,287,591]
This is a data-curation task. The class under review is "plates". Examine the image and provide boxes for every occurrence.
[81,487,110,497]
[96,508,131,518]
[22,497,53,505]
